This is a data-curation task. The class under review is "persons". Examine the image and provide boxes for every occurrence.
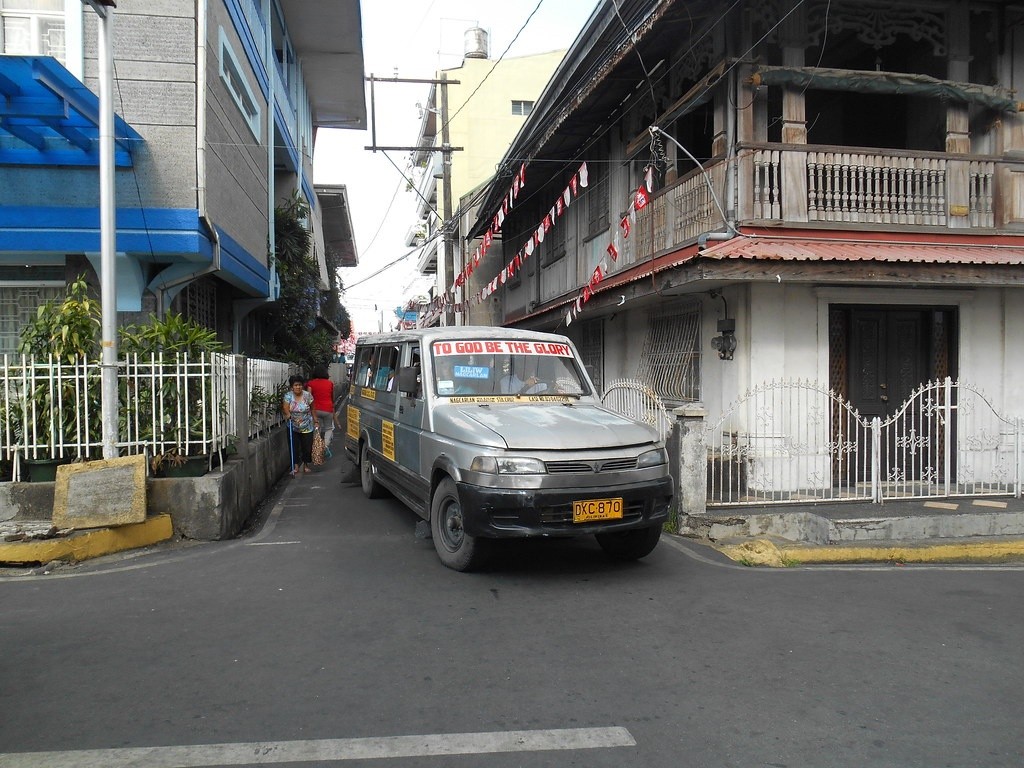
[356,348,420,399]
[301,365,344,458]
[499,355,567,396]
[434,358,477,397]
[331,350,337,363]
[282,375,319,476]
[338,352,346,365]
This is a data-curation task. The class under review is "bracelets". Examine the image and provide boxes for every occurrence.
[314,421,319,424]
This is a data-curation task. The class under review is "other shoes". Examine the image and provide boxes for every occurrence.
[290,470,297,476]
[304,467,311,473]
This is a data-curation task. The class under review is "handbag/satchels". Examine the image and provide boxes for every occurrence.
[311,427,332,466]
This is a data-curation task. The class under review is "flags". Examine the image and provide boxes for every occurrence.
[408,161,656,331]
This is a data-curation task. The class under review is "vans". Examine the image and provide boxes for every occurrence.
[341,326,674,572]
